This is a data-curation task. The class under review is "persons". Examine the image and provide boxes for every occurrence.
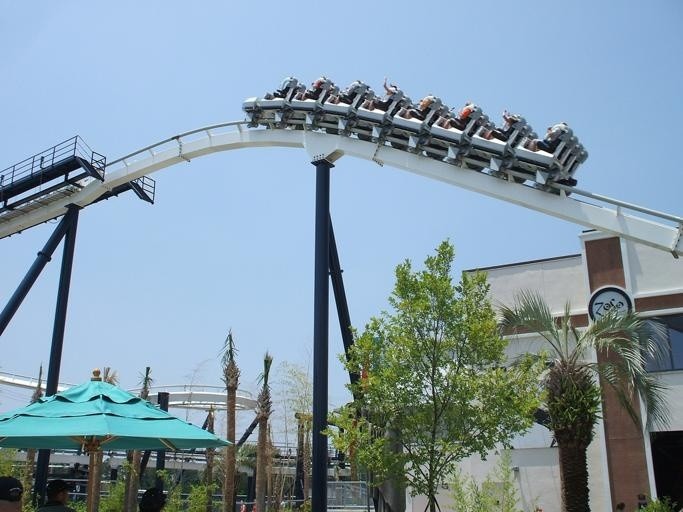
[138,486,165,511]
[35,478,75,510]
[279,496,285,509]
[239,499,247,512]
[0,474,24,512]
[252,498,256,512]
[263,76,568,153]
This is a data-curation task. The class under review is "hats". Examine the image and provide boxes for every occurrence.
[0,476,24,502]
[46,480,73,496]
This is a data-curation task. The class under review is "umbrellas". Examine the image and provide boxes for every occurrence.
[0,366,234,512]
